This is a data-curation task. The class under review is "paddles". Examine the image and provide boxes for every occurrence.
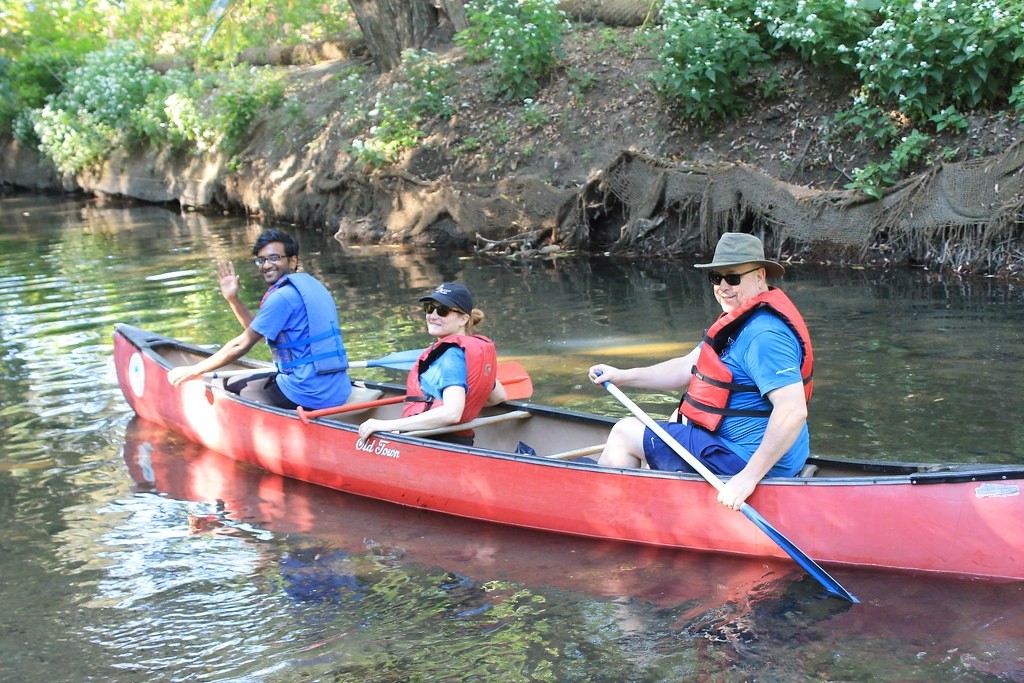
[201,346,437,378]
[594,369,859,602]
[296,361,533,427]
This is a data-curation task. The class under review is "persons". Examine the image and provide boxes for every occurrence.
[165,231,352,413]
[357,282,505,449]
[587,233,812,513]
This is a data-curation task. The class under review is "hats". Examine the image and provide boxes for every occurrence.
[694,232,785,279]
[417,283,473,316]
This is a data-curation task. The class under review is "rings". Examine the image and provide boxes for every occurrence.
[728,505,733,509]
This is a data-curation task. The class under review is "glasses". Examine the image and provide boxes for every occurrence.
[254,255,288,266]
[708,267,766,286]
[423,303,451,316]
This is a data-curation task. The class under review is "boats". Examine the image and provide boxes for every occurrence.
[113,321,1024,584]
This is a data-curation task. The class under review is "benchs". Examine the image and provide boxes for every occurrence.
[291,387,384,419]
